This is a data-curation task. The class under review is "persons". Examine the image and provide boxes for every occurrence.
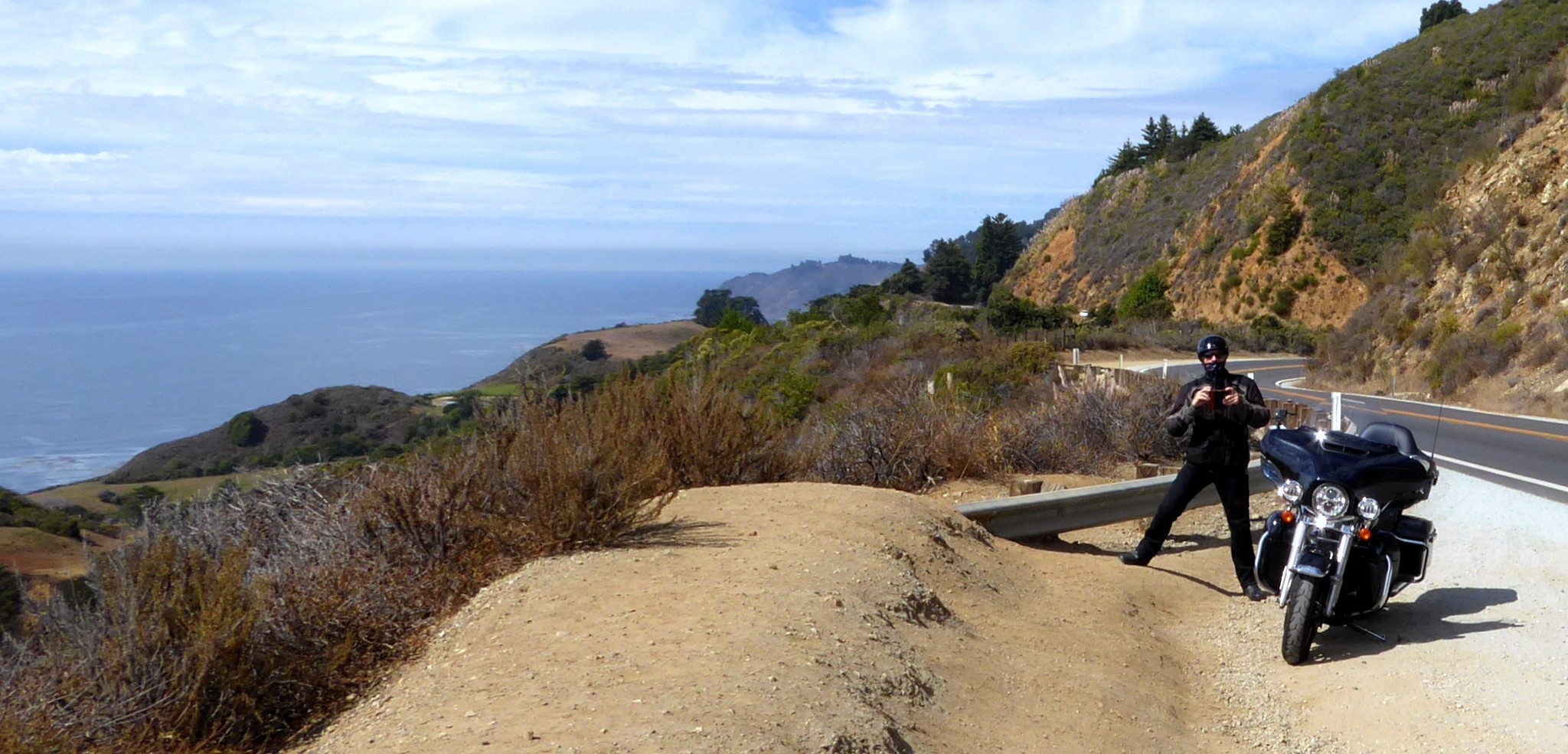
[1116,334,1271,601]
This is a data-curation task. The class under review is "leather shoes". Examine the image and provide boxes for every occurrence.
[1119,550,1146,566]
[1242,583,1267,601]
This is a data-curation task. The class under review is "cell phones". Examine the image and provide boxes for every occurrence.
[1209,390,1231,410]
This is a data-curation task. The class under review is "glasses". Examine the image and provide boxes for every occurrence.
[1203,352,1222,359]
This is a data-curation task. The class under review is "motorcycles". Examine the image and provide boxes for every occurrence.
[1254,393,1443,666]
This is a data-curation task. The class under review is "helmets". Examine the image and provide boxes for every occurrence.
[1197,334,1229,356]
